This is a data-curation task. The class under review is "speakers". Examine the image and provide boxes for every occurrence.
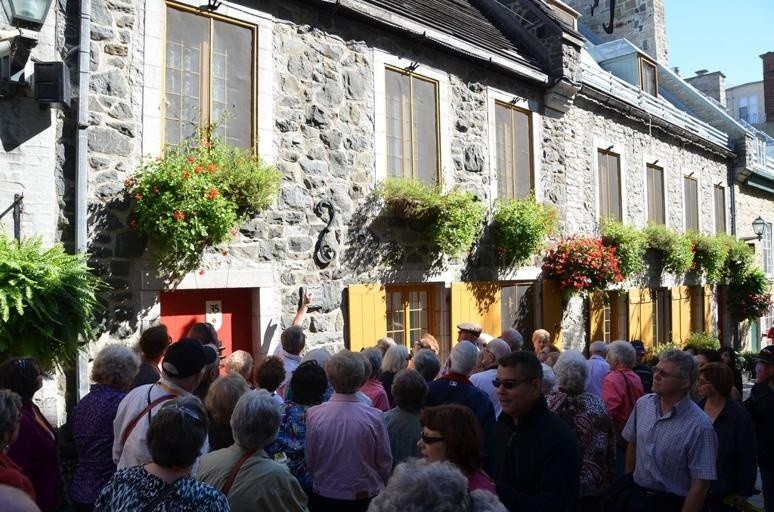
[34,62,71,110]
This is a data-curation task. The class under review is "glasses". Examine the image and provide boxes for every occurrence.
[421,432,441,444]
[492,381,521,389]
[652,366,688,380]
[161,404,199,421]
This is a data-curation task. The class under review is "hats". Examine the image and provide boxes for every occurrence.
[457,322,482,337]
[752,346,773,364]
[162,338,216,378]
[631,340,646,354]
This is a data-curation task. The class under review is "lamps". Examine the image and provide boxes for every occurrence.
[1,1,54,99]
[738,215,765,244]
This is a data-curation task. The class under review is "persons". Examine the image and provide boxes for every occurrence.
[0,283,774,512]
